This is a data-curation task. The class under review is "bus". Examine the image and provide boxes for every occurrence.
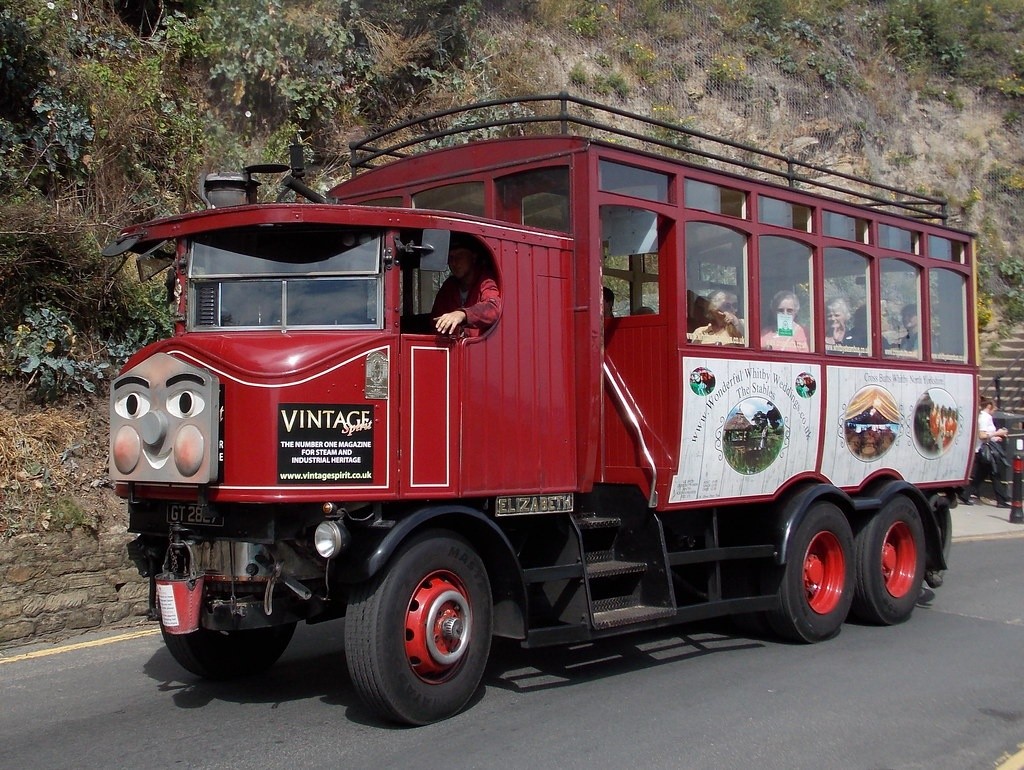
[106,93,987,727]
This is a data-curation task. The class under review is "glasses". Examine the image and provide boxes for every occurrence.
[722,303,739,309]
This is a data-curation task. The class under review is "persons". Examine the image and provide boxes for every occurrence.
[424,239,503,335]
[602,286,920,357]
[958,395,1012,508]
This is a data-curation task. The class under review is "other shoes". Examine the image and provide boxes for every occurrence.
[970,493,998,506]
[997,502,1011,508]
[958,494,974,505]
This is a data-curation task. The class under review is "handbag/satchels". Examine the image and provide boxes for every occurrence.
[980,434,992,464]
